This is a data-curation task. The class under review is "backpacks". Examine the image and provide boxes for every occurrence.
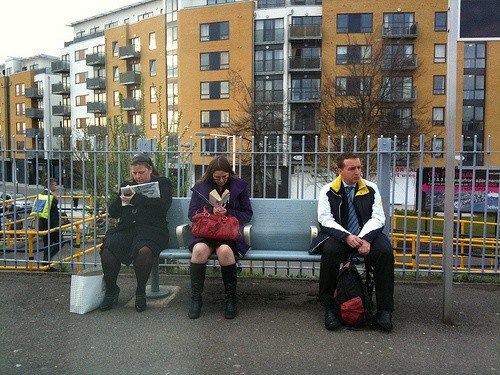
[329,258,376,329]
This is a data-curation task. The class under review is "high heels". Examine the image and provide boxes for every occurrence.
[99,284,120,310]
[135,288,146,312]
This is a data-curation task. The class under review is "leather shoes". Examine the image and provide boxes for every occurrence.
[324,307,342,331]
[374,309,393,331]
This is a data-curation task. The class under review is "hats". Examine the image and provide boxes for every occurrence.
[45,178,58,182]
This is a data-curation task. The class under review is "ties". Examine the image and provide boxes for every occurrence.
[345,186,360,236]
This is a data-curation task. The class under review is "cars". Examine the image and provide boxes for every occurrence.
[0,192,32,214]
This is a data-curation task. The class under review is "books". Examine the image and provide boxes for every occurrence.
[209,188,230,209]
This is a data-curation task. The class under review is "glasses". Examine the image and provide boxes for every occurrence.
[129,156,150,164]
[211,174,231,182]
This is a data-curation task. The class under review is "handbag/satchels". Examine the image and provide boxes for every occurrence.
[190,203,240,241]
[30,193,55,219]
[69,274,106,314]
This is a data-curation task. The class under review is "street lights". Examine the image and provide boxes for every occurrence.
[195,132,236,173]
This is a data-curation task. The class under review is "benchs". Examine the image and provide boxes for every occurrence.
[144,196,371,310]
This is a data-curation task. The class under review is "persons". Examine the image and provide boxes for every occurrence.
[307,152,395,332]
[42,178,64,271]
[187,156,252,318]
[98,153,173,311]
[73,195,79,210]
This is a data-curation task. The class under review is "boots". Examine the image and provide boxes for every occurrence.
[220,263,237,319]
[187,262,207,319]
